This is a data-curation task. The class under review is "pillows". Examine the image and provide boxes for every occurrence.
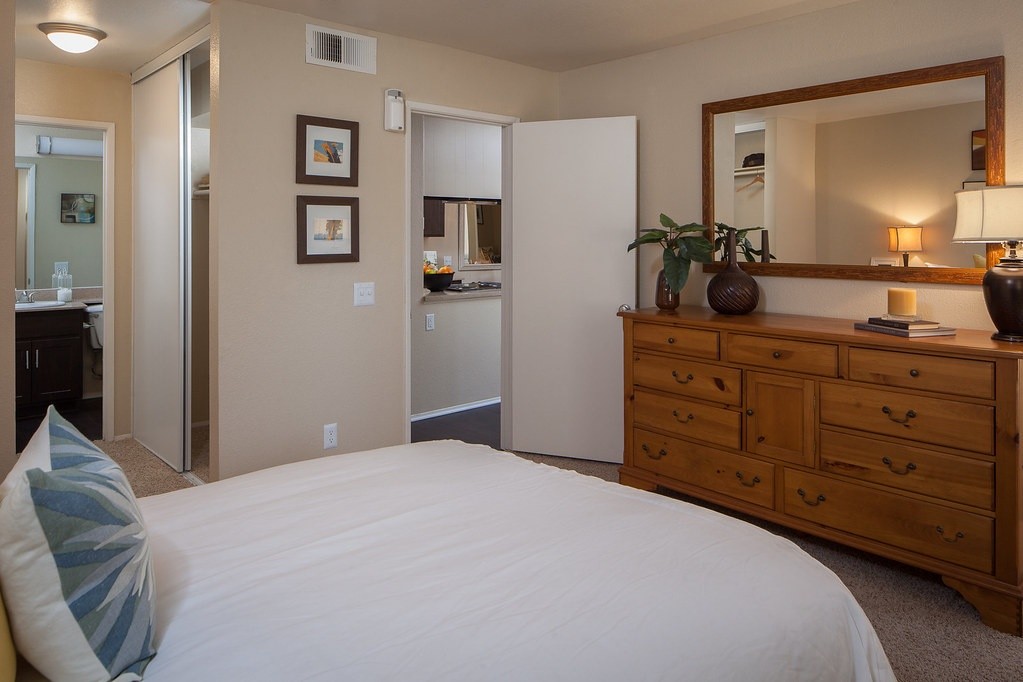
[973,253,985,268]
[0,404,156,682]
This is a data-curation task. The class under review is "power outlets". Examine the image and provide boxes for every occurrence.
[425,314,434,331]
[324,423,338,450]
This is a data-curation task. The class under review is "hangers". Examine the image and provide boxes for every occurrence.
[736,169,764,193]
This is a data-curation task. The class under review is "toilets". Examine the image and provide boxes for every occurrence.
[86,304,103,349]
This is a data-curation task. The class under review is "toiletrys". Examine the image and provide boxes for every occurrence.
[52,268,59,288]
[56,268,73,302]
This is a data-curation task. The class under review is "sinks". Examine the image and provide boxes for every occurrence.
[15,301,66,309]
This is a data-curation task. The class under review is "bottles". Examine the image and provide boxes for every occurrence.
[52,266,58,288]
[57,266,72,301]
[707,229,759,315]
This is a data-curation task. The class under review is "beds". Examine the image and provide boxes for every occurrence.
[0,438,898,682]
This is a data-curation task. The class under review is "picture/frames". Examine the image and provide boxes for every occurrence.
[297,195,359,264]
[963,182,986,190]
[296,114,359,187]
[60,193,95,223]
[871,257,899,267]
[971,129,986,170]
[476,205,483,224]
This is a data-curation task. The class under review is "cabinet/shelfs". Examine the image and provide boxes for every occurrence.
[15,309,86,452]
[614,304,1022,636]
[735,116,816,263]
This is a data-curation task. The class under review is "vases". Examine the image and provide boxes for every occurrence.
[707,228,760,315]
[761,230,770,265]
[655,269,679,312]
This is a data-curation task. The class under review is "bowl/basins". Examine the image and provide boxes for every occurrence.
[424,272,455,292]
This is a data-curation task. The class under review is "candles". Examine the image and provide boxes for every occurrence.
[888,288,916,318]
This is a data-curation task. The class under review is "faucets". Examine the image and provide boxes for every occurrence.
[16,290,36,303]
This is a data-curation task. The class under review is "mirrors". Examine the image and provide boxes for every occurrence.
[458,203,502,271]
[701,56,1007,286]
[15,163,35,291]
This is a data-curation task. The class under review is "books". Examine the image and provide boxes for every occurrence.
[853,314,957,338]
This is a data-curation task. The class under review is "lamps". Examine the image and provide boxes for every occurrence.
[951,183,1023,342]
[887,226,924,267]
[36,23,107,54]
[37,135,103,158]
